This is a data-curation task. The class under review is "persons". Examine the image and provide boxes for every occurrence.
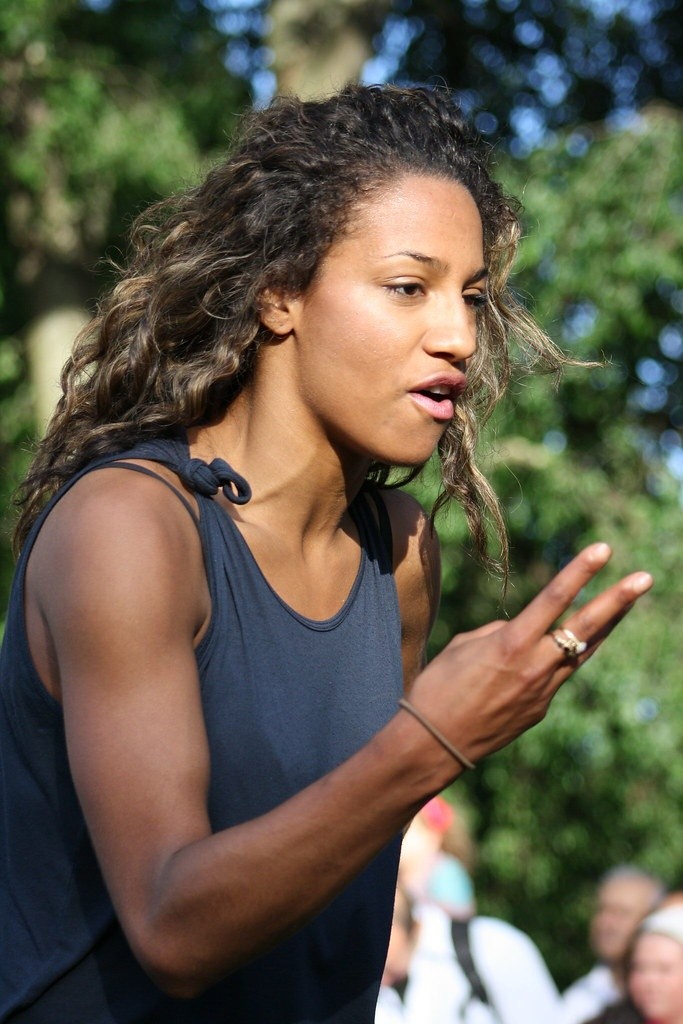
[373,791,683,1024]
[0,80,654,1024]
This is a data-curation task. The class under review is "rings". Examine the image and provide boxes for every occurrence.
[550,628,590,665]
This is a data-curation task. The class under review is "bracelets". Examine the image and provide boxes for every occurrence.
[397,695,479,769]
